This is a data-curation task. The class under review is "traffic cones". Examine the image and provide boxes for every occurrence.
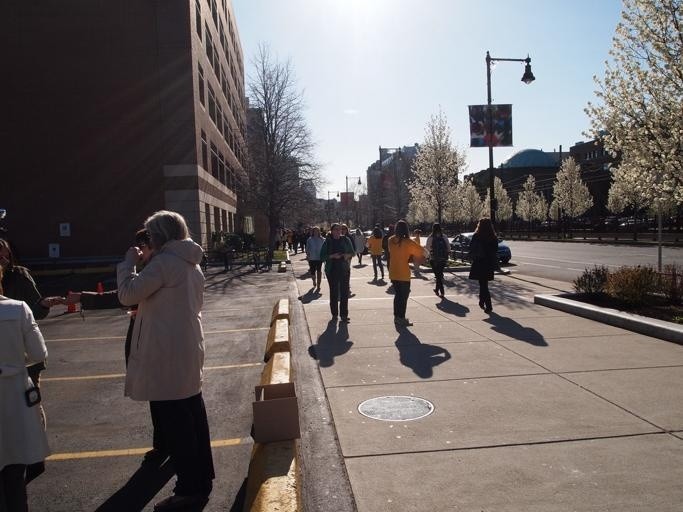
[95,280,104,294]
[65,291,79,313]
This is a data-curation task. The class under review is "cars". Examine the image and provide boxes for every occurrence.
[572,216,592,234]
[448,233,511,265]
[540,219,569,235]
[605,215,655,233]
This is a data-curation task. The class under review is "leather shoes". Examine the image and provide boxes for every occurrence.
[154,493,208,510]
[144,448,160,458]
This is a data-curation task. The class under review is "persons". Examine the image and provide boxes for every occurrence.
[387,219,430,325]
[0,237,65,476]
[115,210,217,512]
[319,222,357,323]
[466,217,499,313]
[65,228,155,370]
[273,222,423,290]
[0,281,49,512]
[424,222,451,297]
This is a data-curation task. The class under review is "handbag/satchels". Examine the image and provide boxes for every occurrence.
[362,247,368,255]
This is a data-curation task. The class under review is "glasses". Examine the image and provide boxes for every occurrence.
[146,231,159,239]
[137,241,146,249]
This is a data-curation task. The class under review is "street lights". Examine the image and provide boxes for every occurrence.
[345,176,361,221]
[486,50,535,232]
[327,191,340,226]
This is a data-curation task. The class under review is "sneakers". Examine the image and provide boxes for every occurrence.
[332,316,350,321]
[479,301,492,313]
[394,317,413,326]
[433,288,444,298]
[348,293,355,298]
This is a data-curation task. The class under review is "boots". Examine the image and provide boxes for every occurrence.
[317,272,321,290]
[311,273,316,286]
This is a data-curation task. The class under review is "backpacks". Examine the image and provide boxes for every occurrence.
[431,233,448,260]
